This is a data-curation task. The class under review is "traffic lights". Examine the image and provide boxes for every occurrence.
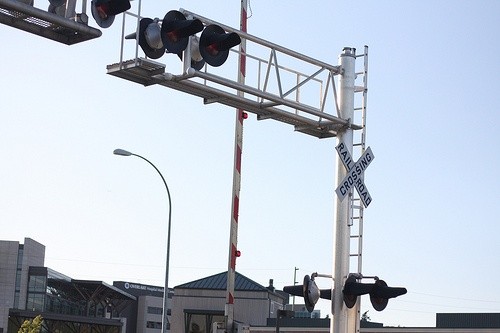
[91,0,131,27]
[343,273,407,311]
[283,274,321,314]
[317,287,336,315]
[198,24,241,67]
[161,10,204,53]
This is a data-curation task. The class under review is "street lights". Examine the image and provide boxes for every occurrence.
[112,148,172,333]
